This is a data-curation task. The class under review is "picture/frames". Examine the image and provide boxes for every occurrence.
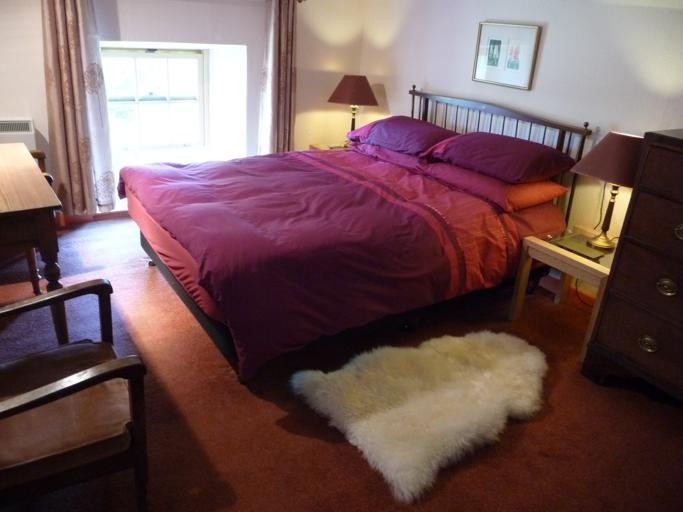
[468,20,543,92]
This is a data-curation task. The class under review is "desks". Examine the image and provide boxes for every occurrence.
[505,222,620,367]
[0,141,68,346]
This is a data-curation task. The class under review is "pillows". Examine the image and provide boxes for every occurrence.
[415,163,571,214]
[343,113,457,155]
[419,125,574,178]
[346,143,428,176]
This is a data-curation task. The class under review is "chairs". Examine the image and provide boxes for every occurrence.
[0,279,150,509]
[27,148,53,187]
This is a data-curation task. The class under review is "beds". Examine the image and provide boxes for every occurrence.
[113,82,593,384]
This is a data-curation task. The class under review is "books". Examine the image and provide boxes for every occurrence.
[552,232,607,258]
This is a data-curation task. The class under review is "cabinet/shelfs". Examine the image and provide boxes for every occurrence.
[579,127,682,409]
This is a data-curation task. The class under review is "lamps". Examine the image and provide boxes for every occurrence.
[323,70,379,146]
[568,126,646,251]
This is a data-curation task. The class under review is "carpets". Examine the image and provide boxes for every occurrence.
[283,322,551,505]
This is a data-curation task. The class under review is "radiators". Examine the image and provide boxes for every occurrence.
[0,119,38,151]
